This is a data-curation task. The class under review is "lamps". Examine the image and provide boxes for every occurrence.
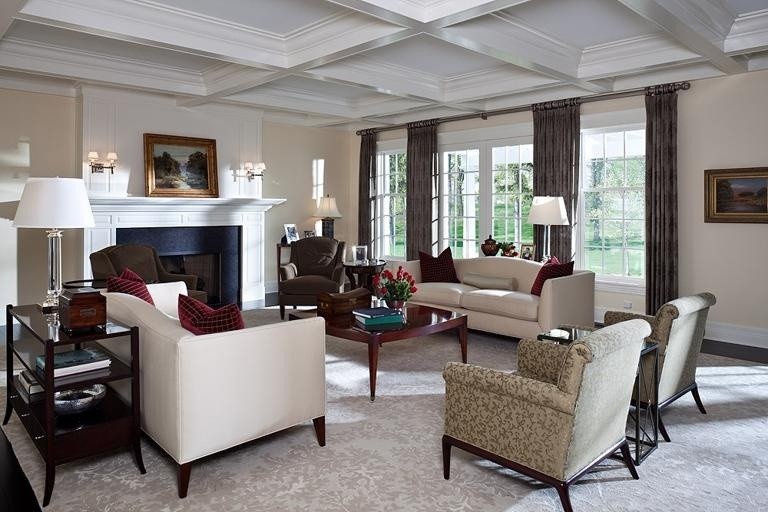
[87,149,118,177]
[10,175,97,317]
[311,192,343,241]
[526,193,571,264]
[242,161,266,184]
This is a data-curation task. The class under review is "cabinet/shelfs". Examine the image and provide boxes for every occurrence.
[2,297,147,507]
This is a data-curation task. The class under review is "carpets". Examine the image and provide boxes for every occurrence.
[0,301,765,512]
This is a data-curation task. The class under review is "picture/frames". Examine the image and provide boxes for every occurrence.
[519,243,537,263]
[282,222,300,248]
[351,244,369,264]
[141,131,220,199]
[701,166,768,226]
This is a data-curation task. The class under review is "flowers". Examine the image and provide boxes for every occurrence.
[497,240,519,258]
[372,262,418,303]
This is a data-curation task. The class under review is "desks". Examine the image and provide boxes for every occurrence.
[284,292,470,403]
[341,257,388,293]
[275,243,292,297]
[532,322,664,467]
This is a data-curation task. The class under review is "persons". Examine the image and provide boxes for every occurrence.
[522,248,532,259]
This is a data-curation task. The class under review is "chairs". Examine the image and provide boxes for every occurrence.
[273,234,347,322]
[437,316,656,512]
[86,242,210,311]
[554,286,721,444]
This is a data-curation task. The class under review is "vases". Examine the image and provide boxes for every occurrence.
[384,299,407,310]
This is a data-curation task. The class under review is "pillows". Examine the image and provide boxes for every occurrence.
[104,266,156,309]
[176,292,248,338]
[528,254,575,298]
[459,270,519,293]
[416,246,461,284]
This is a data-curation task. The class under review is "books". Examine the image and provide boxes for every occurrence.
[18,346,111,393]
[352,307,403,331]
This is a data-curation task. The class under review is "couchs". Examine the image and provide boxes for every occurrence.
[82,275,331,502]
[385,252,598,347]
